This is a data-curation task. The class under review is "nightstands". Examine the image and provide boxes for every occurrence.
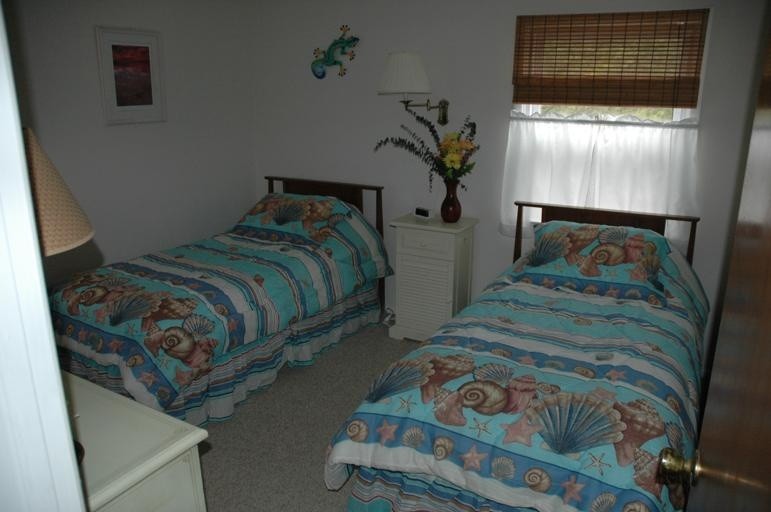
[388,212,481,343]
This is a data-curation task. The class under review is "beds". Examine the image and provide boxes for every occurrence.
[47,177,394,428]
[323,202,710,512]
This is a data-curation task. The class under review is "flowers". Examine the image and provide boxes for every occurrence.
[374,108,480,192]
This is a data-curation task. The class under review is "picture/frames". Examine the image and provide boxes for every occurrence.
[95,25,168,125]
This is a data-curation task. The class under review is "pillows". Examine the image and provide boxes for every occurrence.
[225,193,352,248]
[481,220,673,308]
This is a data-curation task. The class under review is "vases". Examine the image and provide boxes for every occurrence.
[441,180,461,223]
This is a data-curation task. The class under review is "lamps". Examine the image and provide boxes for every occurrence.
[23,128,97,465]
[375,52,449,127]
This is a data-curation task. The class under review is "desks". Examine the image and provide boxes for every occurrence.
[62,370,209,512]
